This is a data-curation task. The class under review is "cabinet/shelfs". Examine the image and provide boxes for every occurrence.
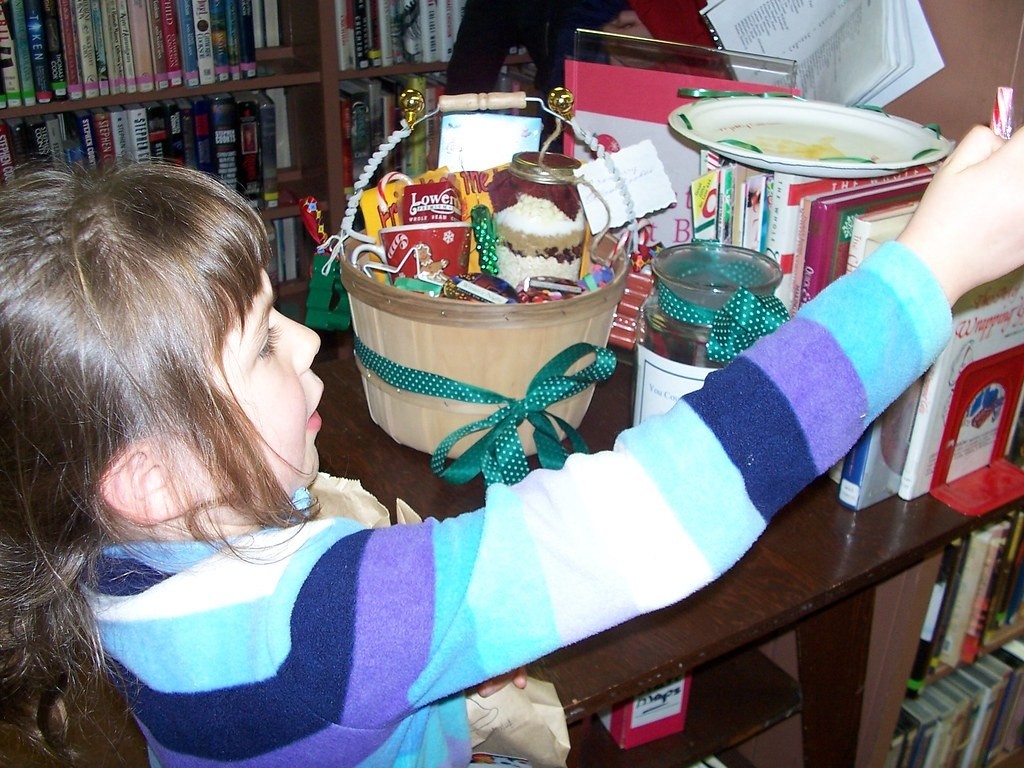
[0,0,536,286]
[889,508,1022,768]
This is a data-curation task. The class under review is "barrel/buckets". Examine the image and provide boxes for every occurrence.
[326,81,632,464]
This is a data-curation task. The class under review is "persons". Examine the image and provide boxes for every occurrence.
[0,121,1024,768]
[424,0,713,173]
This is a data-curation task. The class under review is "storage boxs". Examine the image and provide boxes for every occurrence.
[599,673,691,748]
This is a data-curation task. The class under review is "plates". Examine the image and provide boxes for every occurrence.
[667,97,953,177]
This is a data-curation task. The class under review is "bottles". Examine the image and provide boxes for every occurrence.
[630,243,788,430]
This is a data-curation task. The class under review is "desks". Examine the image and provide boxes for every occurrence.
[274,291,1024,768]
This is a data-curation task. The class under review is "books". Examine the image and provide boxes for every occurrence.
[0,0,466,286]
[884,508,1024,768]
[695,145,1024,512]
[691,755,728,768]
